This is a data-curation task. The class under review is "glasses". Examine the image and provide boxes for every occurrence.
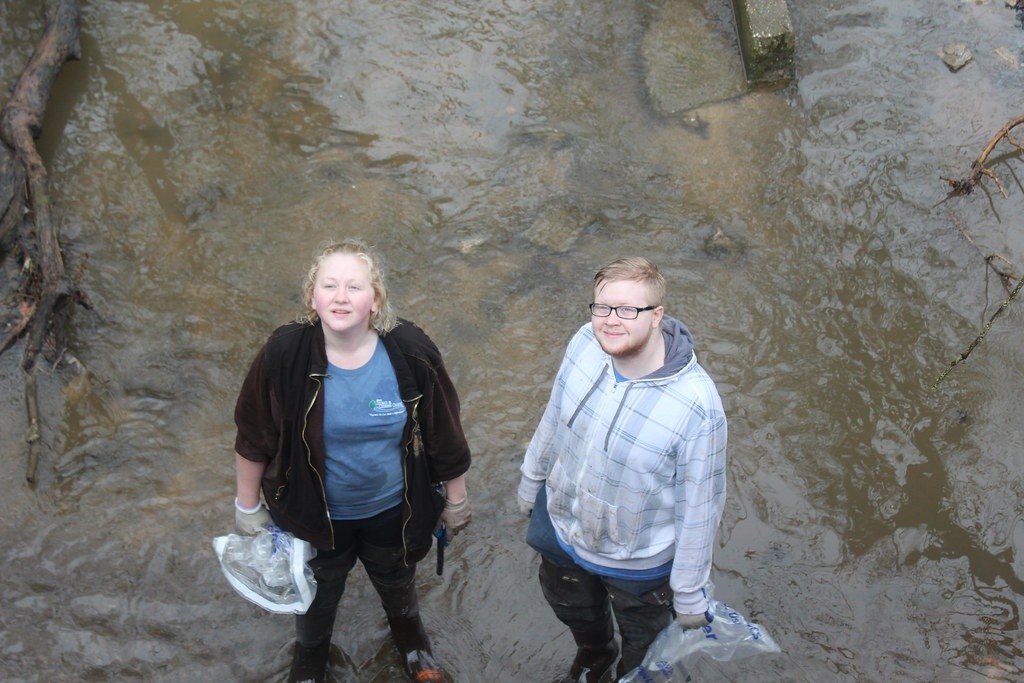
[589,304,657,320]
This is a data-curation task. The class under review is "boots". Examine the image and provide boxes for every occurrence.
[289,638,330,683]
[382,604,444,683]
[568,615,620,683]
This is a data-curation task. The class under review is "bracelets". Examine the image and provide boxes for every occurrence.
[235,497,262,514]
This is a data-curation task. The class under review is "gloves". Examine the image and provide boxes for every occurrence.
[233,496,274,535]
[516,494,536,516]
[433,491,472,546]
[677,611,713,629]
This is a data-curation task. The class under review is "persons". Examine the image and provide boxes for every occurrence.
[516,257,728,683]
[235,243,472,683]
